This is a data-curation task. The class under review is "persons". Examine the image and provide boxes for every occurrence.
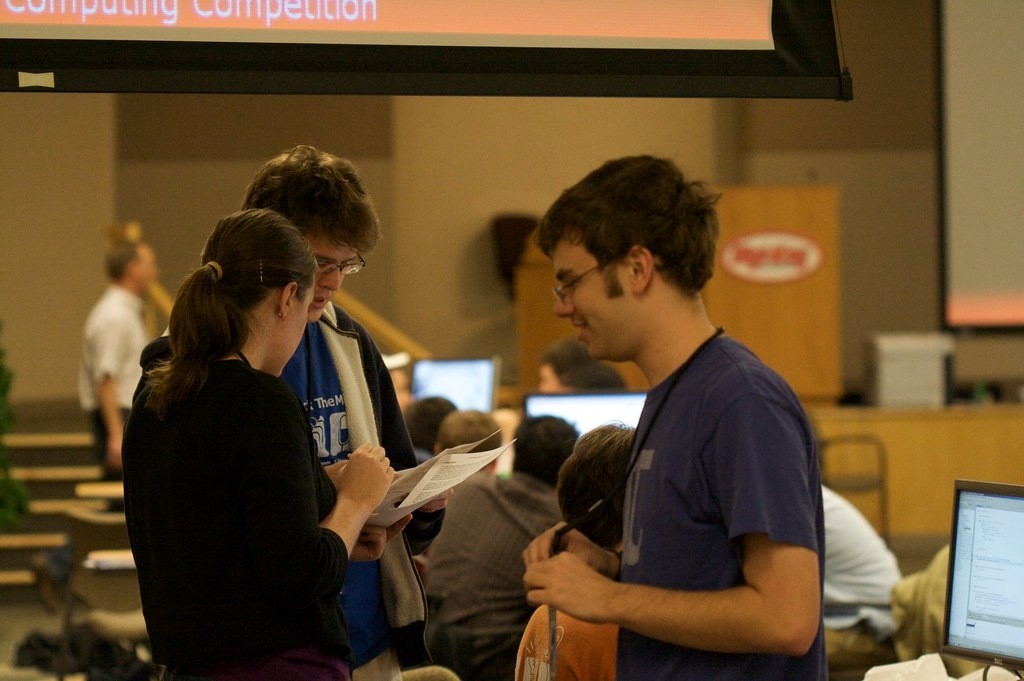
[120,145,453,681]
[522,153,830,681]
[394,337,903,681]
[78,237,157,512]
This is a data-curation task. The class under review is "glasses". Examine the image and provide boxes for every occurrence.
[552,249,662,303]
[317,248,366,275]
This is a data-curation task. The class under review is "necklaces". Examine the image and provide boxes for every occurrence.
[237,350,251,369]
[304,323,312,413]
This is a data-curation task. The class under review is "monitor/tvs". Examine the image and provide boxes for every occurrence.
[524,390,646,440]
[410,355,500,414]
[940,477,1024,672]
